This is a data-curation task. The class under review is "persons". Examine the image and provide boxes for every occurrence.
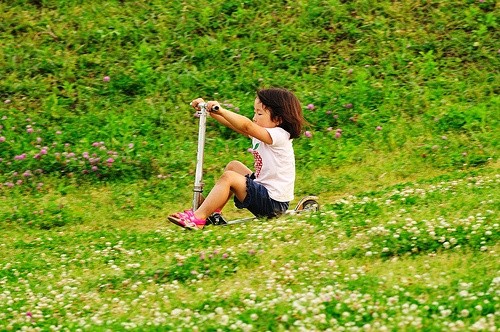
[168,87,318,232]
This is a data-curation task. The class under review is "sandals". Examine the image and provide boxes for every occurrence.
[168,208,206,231]
